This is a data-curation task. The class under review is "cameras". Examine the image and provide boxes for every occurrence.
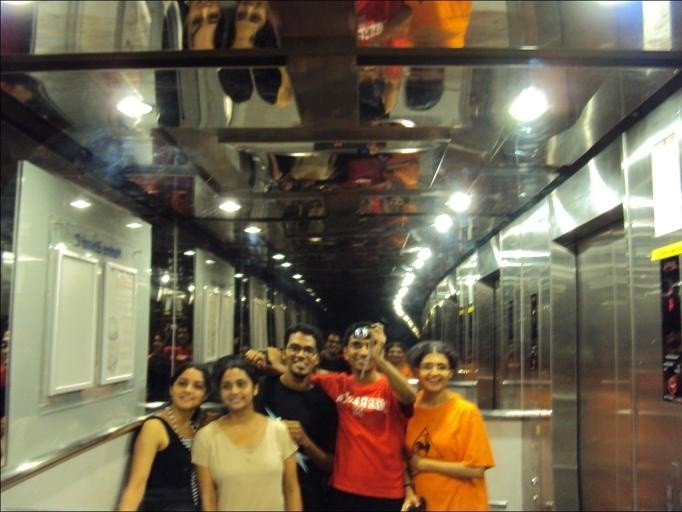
[355,326,370,338]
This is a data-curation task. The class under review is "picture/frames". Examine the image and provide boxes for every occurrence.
[48,248,96,398]
[201,284,268,365]
[99,260,138,387]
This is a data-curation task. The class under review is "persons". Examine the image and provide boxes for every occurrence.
[145,314,194,402]
[0,329,11,469]
[116,317,495,511]
